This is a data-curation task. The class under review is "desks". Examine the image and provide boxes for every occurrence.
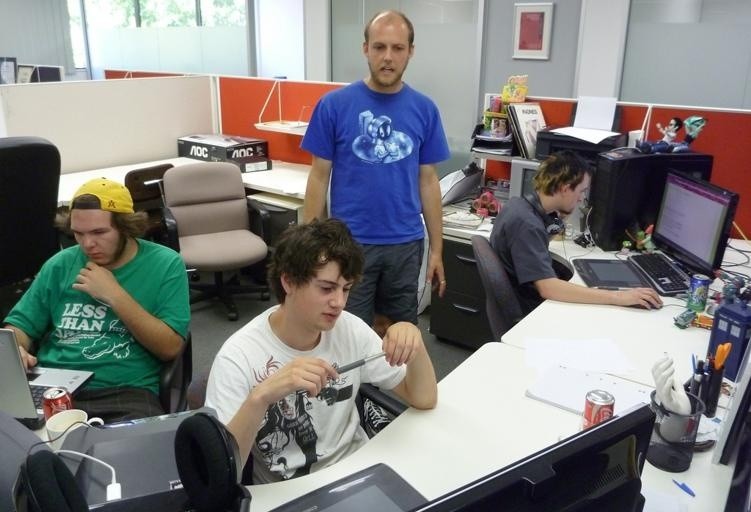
[57,152,329,213]
[245,195,750,512]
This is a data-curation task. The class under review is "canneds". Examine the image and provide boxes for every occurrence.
[686,274,709,311]
[582,390,615,431]
[42,385,72,418]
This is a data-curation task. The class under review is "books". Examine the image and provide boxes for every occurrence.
[502,102,547,160]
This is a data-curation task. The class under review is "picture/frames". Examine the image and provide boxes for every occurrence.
[511,3,553,60]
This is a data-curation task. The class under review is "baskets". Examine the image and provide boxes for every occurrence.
[644,389,706,472]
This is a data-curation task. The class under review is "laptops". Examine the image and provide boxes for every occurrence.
[0,327,96,431]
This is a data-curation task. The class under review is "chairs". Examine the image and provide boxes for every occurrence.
[0,136,60,289]
[471,235,523,342]
[160,331,192,414]
[161,162,271,321]
[124,162,202,282]
[355,382,409,439]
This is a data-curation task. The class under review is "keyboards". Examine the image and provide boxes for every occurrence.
[627,252,692,297]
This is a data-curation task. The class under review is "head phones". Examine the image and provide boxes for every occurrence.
[522,192,565,235]
[11,411,253,512]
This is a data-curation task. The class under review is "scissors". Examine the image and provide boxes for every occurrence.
[714,342,732,370]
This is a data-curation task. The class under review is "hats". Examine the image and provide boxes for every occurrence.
[69,178,135,214]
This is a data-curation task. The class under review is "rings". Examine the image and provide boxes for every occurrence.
[439,280,446,285]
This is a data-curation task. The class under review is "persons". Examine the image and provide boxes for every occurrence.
[636,117,683,154]
[650,352,692,443]
[490,149,663,311]
[2,177,192,425]
[299,9,453,437]
[204,216,438,486]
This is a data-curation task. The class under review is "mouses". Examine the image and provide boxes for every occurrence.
[634,299,663,309]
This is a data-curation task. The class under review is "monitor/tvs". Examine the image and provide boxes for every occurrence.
[650,167,740,280]
[407,402,657,512]
[509,158,586,232]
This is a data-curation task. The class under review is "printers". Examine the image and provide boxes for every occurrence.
[535,103,628,162]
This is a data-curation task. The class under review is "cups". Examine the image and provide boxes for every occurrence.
[46,409,104,451]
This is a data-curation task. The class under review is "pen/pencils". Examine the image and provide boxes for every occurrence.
[336,352,386,374]
[692,353,713,384]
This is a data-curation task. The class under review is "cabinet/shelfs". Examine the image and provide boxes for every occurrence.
[429,237,486,347]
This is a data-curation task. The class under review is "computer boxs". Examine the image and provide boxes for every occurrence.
[591,146,714,252]
